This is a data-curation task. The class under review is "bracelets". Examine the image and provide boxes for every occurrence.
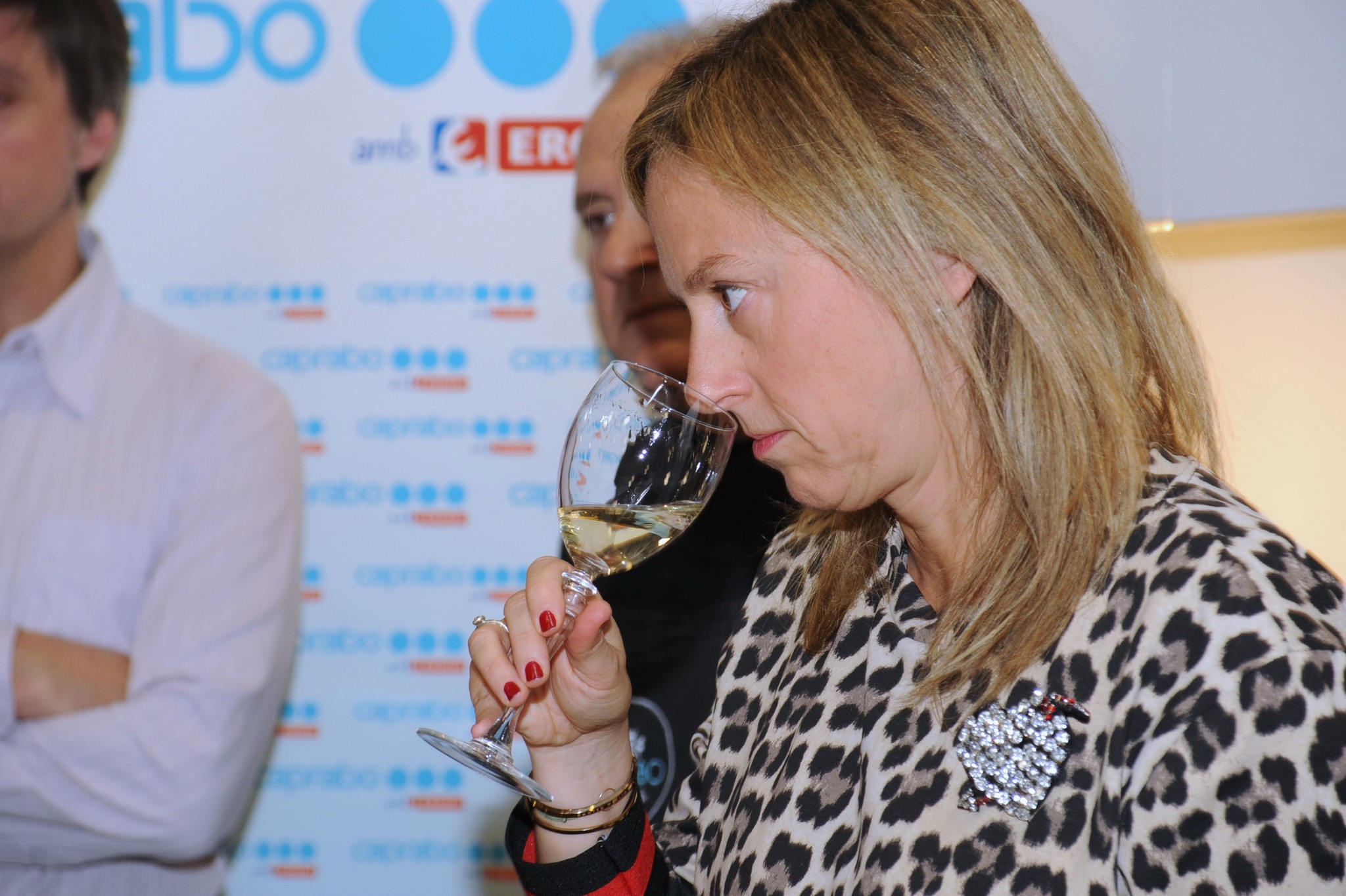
[523,752,638,834]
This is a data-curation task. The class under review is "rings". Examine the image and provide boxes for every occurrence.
[472,615,511,656]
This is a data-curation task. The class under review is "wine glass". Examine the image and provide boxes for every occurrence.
[414,358,743,801]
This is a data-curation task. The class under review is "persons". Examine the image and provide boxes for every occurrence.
[469,2,1345,896]
[560,9,803,844]
[1,0,303,895]
